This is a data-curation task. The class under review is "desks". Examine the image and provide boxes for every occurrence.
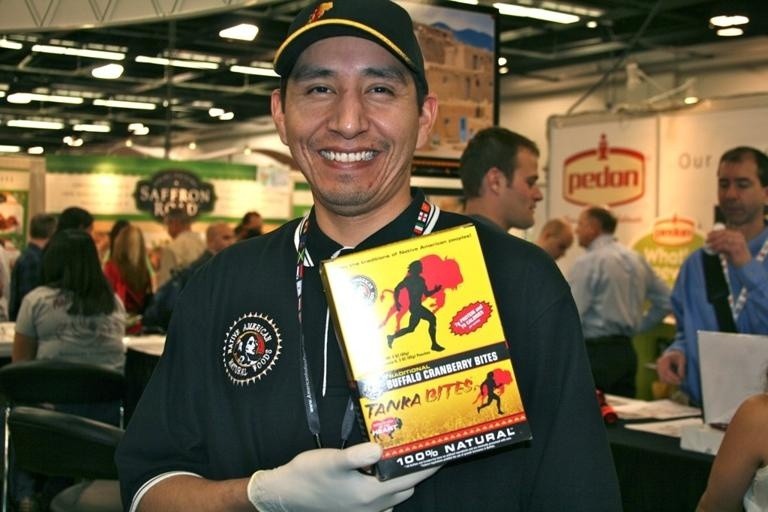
[125,346,715,512]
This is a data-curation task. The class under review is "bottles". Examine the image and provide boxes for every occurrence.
[596,387,617,426]
[704,222,727,256]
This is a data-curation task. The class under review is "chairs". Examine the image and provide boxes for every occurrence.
[3,407,125,512]
[0,362,125,481]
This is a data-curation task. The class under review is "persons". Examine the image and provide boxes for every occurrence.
[477,372,504,415]
[12,228,126,512]
[461,128,543,232]
[695,363,768,512]
[537,218,573,261]
[569,207,676,398]
[234,333,264,364]
[1,202,263,333]
[115,0,623,512]
[656,147,768,411]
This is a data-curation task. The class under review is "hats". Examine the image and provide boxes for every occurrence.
[271,1,430,92]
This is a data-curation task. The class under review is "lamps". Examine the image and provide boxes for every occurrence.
[626,63,669,94]
